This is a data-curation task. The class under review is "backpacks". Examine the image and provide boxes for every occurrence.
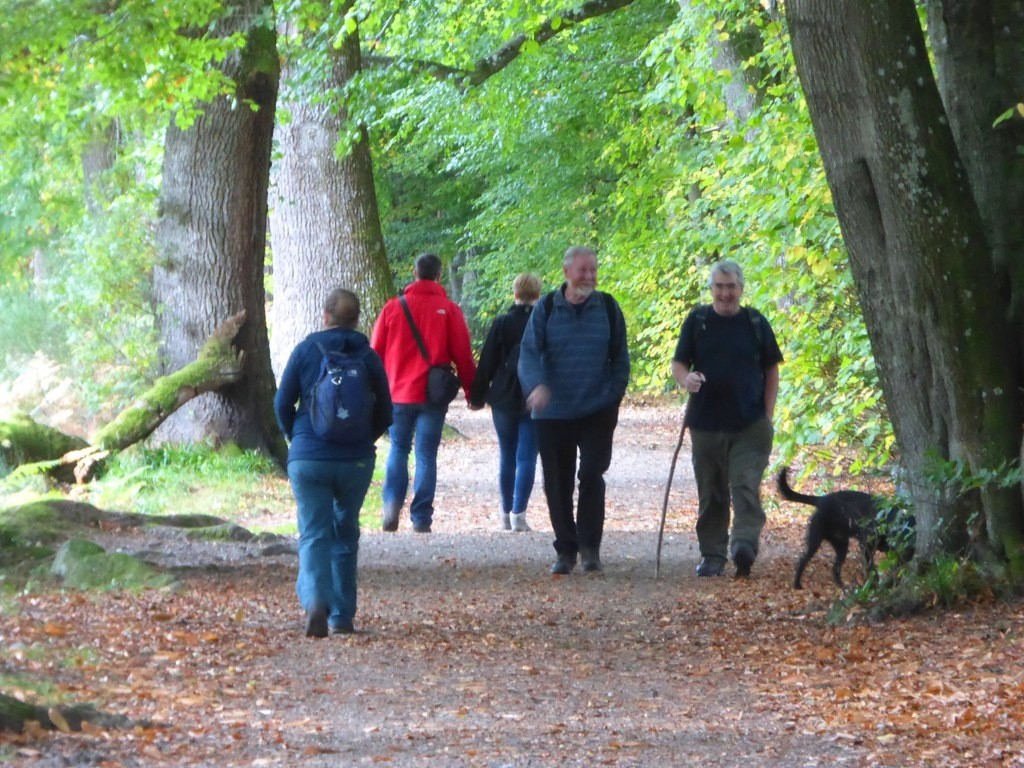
[305,332,376,445]
[483,341,526,411]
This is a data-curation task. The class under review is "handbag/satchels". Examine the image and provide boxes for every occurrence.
[426,365,462,406]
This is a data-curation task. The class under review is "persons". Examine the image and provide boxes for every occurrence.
[673,261,784,577]
[370,254,479,533]
[273,288,394,637]
[469,274,541,531]
[518,246,629,574]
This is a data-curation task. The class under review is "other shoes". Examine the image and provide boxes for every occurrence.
[581,560,602,571]
[509,510,532,531]
[383,516,399,532]
[302,622,329,637]
[414,528,432,532]
[550,559,571,574]
[734,565,751,577]
[694,555,727,577]
[326,623,354,633]
[500,511,511,529]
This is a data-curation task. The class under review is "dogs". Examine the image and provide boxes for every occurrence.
[774,463,916,590]
